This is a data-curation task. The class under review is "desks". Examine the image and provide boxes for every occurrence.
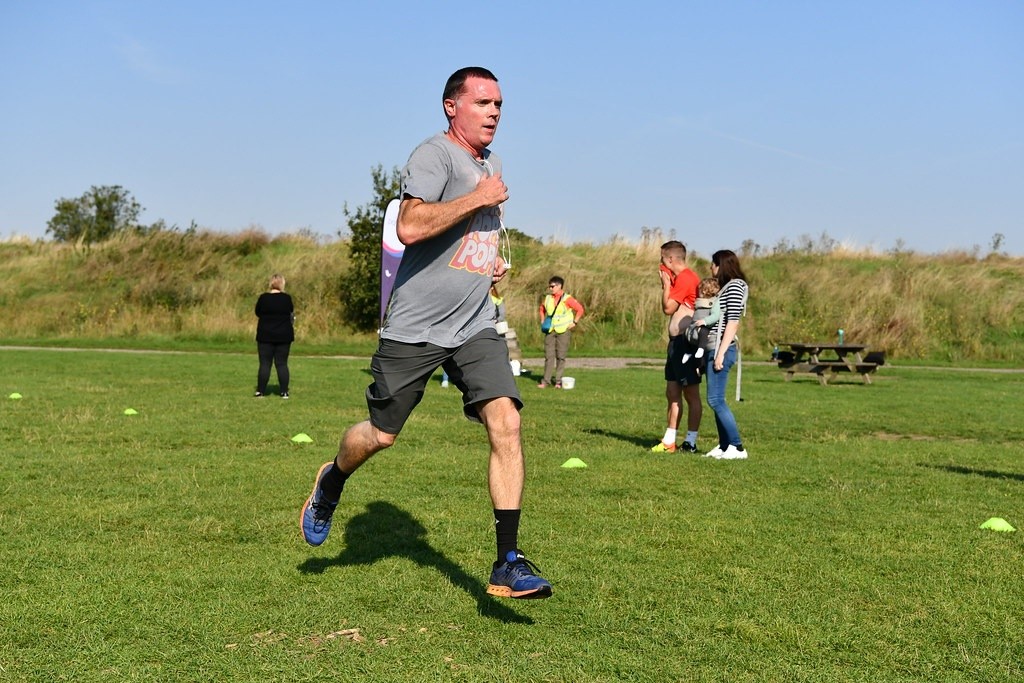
[778,342,872,385]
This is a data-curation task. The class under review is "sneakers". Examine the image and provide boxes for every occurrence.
[487,548,552,599]
[701,444,724,459]
[717,444,748,459]
[677,440,697,454]
[300,461,343,547]
[651,440,676,453]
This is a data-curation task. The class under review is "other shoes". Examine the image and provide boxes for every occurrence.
[538,384,546,388]
[441,380,448,387]
[555,383,561,389]
[280,391,289,398]
[254,391,264,397]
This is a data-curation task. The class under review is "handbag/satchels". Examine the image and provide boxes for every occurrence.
[541,315,552,334]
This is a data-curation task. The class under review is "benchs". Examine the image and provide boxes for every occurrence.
[764,359,876,366]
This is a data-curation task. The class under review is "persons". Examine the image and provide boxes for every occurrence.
[539,276,584,388]
[252,274,295,399]
[703,249,748,459]
[647,240,702,454]
[441,370,449,388]
[488,284,505,324]
[300,67,553,599]
[680,278,720,362]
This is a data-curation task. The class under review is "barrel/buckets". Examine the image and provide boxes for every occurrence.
[561,377,575,389]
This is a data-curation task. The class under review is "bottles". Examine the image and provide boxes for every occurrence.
[838,326,844,346]
[774,346,779,359]
